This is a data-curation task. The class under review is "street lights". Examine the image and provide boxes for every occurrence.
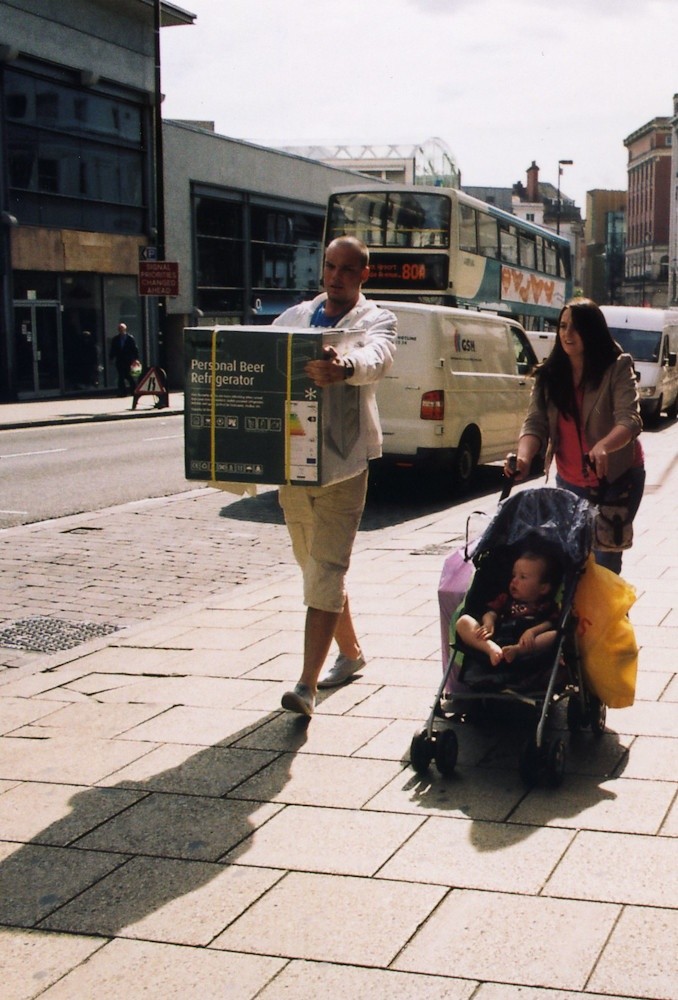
[556,160,574,235]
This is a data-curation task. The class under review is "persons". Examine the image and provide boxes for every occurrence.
[503,297,646,575]
[454,548,565,665]
[83,331,101,390]
[108,322,140,398]
[271,235,398,715]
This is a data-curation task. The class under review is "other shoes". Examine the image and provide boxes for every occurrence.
[117,393,127,397]
[154,402,169,408]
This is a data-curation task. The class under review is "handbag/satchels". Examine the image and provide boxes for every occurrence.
[572,551,637,708]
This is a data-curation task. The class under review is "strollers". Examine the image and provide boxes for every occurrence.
[408,451,607,792]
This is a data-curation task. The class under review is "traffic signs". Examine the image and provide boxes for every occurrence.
[138,246,157,261]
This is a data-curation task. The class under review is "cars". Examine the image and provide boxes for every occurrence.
[523,330,558,364]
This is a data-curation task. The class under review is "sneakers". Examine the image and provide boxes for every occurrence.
[281,683,316,714]
[319,649,366,686]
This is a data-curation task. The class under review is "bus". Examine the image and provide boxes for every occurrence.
[317,183,573,329]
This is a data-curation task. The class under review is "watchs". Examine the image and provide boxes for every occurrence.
[344,357,354,378]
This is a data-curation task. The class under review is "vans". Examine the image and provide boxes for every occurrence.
[361,299,547,492]
[598,304,678,427]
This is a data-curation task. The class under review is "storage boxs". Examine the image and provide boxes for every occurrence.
[183,324,368,486]
